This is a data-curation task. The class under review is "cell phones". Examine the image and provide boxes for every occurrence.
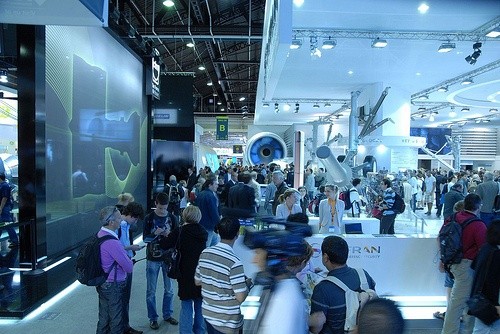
[140,245,146,248]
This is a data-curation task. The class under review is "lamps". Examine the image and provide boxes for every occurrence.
[485,23,500,38]
[290,36,302,49]
[412,78,497,128]
[465,42,482,65]
[371,37,387,48]
[322,36,336,49]
[263,102,332,113]
[310,36,321,58]
[438,40,456,52]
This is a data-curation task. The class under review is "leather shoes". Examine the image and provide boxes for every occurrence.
[150,320,159,330]
[164,316,179,325]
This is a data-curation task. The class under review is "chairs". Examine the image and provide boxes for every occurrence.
[345,223,364,234]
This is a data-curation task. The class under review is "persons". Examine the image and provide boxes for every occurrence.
[95,163,500,334]
[0,173,19,248]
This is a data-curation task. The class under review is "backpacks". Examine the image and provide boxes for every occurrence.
[74,231,120,287]
[166,183,180,204]
[190,184,201,201]
[390,194,406,215]
[438,213,481,265]
[320,267,379,334]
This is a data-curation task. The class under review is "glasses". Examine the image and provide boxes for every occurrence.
[106,207,118,221]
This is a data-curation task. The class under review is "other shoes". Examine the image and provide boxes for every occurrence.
[124,327,144,334]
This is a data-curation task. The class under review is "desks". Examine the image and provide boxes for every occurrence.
[305,217,446,296]
